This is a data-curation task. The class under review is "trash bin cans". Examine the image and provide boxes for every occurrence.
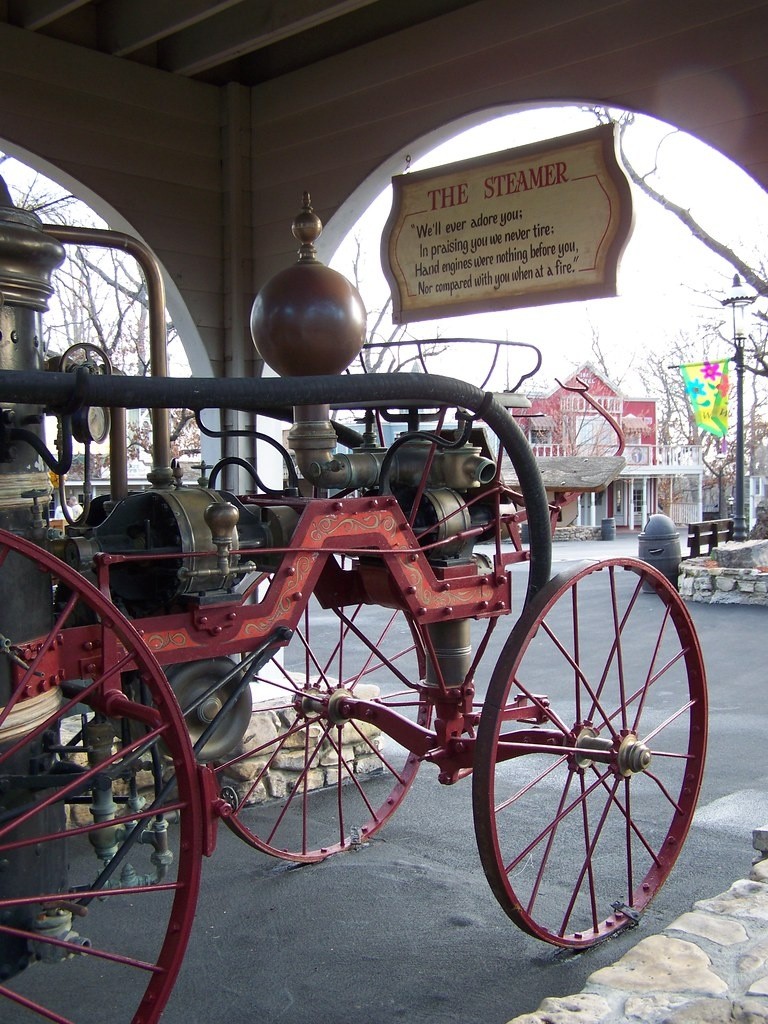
[601,517,616,540]
[637,513,682,594]
[521,523,529,544]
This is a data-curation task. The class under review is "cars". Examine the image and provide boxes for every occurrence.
[0,169,711,1024]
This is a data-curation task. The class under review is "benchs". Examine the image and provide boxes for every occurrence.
[679,518,734,559]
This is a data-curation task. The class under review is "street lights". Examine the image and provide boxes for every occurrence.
[719,274,756,542]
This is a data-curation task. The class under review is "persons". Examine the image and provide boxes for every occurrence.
[55,496,84,522]
